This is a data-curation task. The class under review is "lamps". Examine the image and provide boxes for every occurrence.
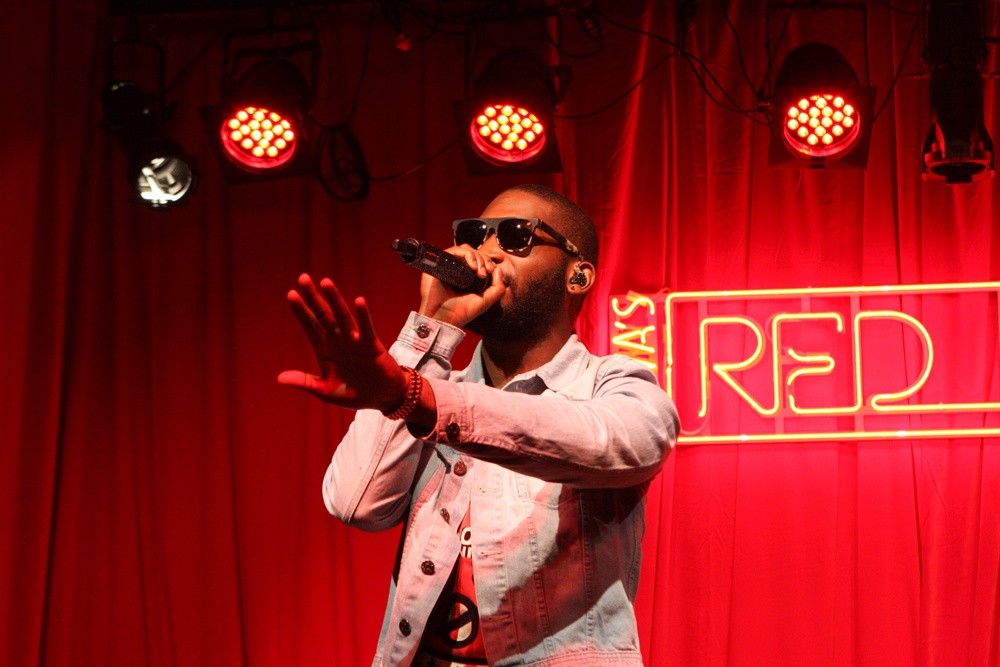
[456,6,572,179]
[764,0,880,173]
[96,78,198,211]
[198,26,325,186]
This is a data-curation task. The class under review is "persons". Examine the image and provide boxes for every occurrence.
[279,185,677,667]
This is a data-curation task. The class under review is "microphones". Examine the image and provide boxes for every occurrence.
[389,235,491,296]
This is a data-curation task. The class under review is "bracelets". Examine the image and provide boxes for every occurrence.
[382,365,422,421]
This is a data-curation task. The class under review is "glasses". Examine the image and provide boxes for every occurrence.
[452,217,583,260]
